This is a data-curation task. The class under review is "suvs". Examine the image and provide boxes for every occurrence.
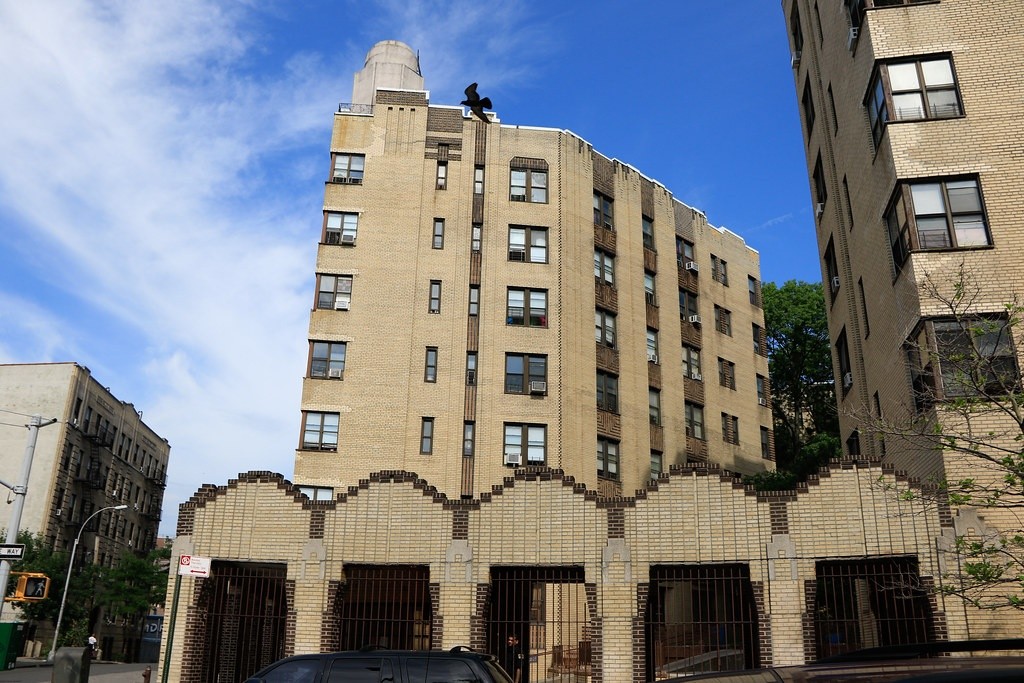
[240,649,515,683]
[640,636,1024,683]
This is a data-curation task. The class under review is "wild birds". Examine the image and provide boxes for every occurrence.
[460,83,492,124]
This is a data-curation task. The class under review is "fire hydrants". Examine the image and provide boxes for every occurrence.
[142,666,152,683]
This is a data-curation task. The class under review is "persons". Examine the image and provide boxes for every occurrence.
[506,636,526,683]
[88,633,97,656]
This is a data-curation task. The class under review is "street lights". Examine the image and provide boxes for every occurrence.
[46,503,128,660]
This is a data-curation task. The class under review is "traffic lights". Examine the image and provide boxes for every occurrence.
[23,575,49,600]
